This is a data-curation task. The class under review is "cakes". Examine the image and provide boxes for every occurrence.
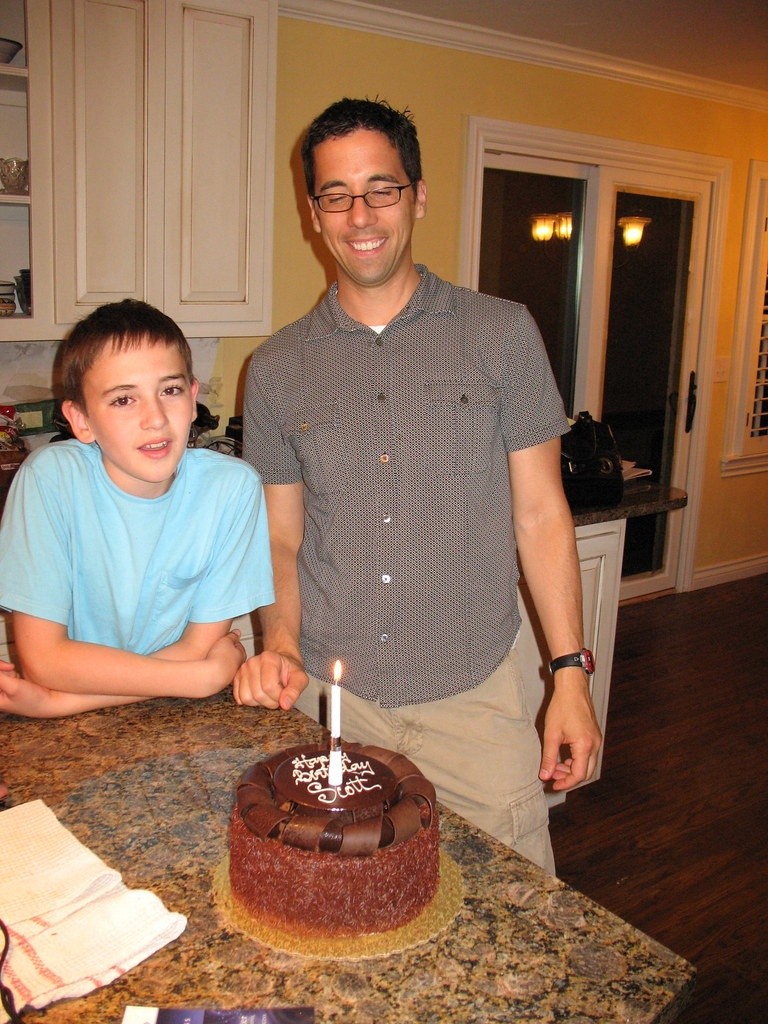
[227,741,442,938]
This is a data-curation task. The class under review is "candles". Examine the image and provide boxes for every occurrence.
[329,660,344,738]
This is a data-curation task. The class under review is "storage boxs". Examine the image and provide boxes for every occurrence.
[0,385,59,436]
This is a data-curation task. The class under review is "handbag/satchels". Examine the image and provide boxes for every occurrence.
[561,411,625,514]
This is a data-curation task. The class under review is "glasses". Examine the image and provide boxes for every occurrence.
[313,181,416,213]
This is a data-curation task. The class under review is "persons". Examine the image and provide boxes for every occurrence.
[0,296,276,719]
[232,94,602,877]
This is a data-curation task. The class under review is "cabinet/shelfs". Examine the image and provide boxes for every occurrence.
[517,518,627,809]
[0,0,274,344]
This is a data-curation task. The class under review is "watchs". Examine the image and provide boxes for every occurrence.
[548,648,596,676]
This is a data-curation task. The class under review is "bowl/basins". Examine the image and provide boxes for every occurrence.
[0,38,23,64]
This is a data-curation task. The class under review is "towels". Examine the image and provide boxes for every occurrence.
[1,800,188,1019]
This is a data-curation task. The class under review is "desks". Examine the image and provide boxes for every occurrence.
[0,692,696,1024]
